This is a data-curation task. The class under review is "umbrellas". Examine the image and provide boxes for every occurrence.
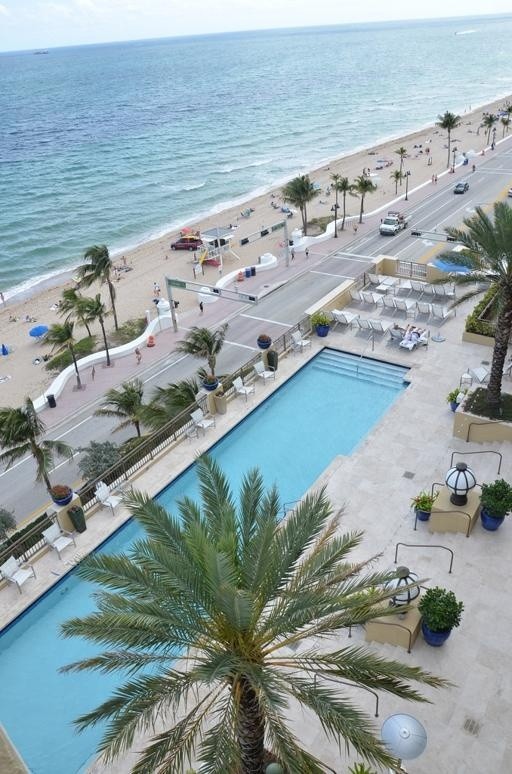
[29,325,49,337]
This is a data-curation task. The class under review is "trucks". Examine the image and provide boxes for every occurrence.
[380,211,411,235]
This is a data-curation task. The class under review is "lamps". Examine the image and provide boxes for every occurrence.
[446,464,476,506]
[385,567,419,619]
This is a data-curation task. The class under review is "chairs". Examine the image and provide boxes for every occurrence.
[42,523,76,559]
[473,355,512,384]
[290,331,310,354]
[0,556,36,593]
[231,376,256,403]
[331,274,456,351]
[189,408,215,438]
[252,360,276,386]
[94,484,124,516]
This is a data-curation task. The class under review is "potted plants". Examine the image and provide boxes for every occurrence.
[200,374,218,392]
[256,334,271,349]
[447,389,468,412]
[50,485,72,506]
[309,312,333,337]
[420,586,462,647]
[411,492,438,522]
[479,480,512,531]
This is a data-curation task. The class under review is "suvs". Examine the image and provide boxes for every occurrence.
[170,236,202,250]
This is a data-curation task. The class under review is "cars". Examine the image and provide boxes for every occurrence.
[454,182,469,194]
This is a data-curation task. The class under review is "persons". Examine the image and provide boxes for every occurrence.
[122,256,127,266]
[304,247,310,261]
[199,301,204,313]
[134,347,143,361]
[40,354,50,362]
[403,324,426,343]
[289,247,297,263]
[152,281,160,298]
[228,102,502,230]
